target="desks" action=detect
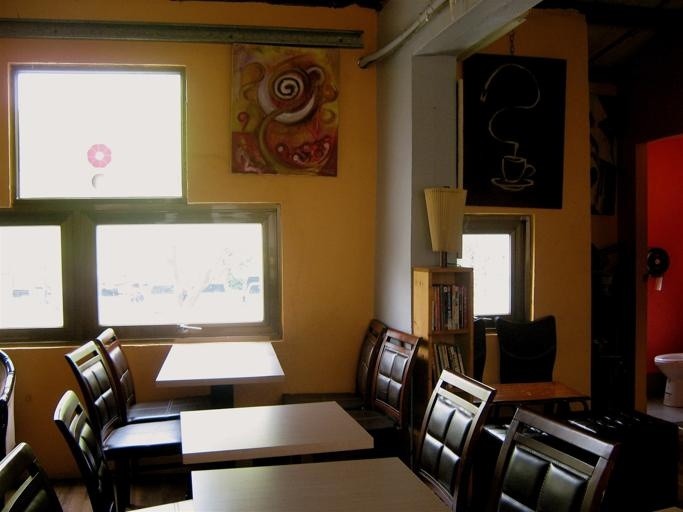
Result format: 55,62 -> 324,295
190,457 -> 450,509
156,339 -> 285,408
180,402 -> 375,500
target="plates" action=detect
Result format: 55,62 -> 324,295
492,176 -> 536,192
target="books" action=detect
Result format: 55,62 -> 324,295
431,282 -> 469,333
431,343 -> 466,390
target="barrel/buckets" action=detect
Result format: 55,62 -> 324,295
654,353 -> 683,408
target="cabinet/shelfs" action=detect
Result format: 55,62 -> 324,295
412,267 -> 476,435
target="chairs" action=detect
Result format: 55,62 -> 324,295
483,408 -> 618,510
0,328 -> 216,512
496,315 -> 592,421
282,322 -> 388,409
411,369 -> 498,510
474,322 -> 487,383
340,328 -> 423,466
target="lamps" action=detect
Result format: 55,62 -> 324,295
424,185 -> 467,269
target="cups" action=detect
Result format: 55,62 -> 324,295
500,154 -> 537,184
